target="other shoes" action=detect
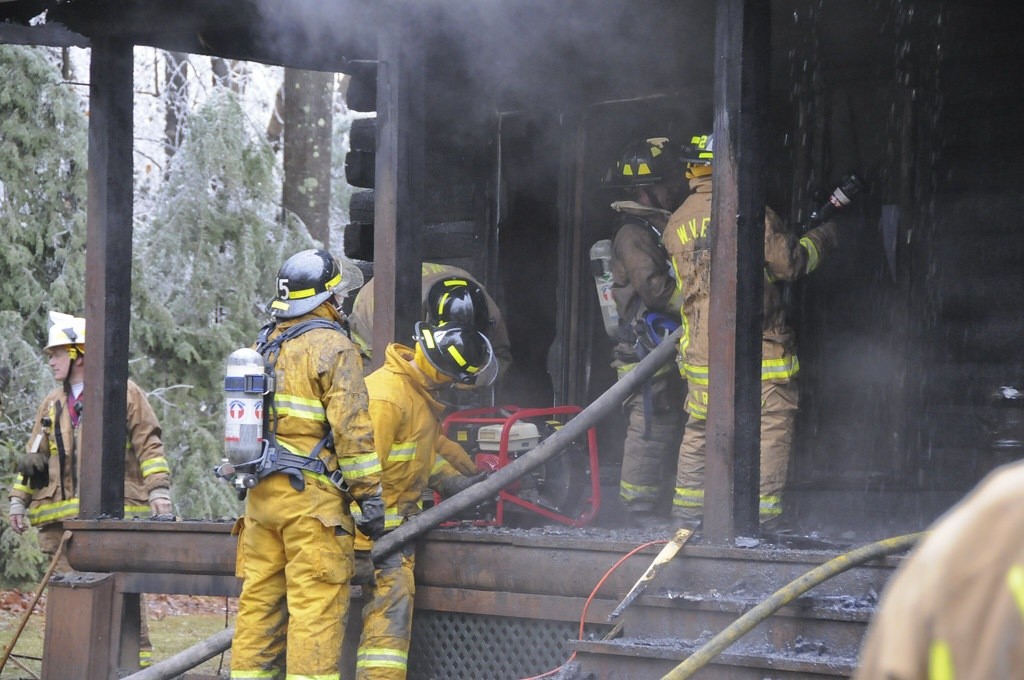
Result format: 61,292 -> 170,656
624,512 -> 676,530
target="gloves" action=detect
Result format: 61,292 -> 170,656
15,454 -> 50,490
436,470 -> 492,515
355,496 -> 385,541
825,207 -> 850,240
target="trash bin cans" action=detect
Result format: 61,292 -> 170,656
984,392 -> 1024,469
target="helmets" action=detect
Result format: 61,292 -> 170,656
413,321 -> 498,388
679,133 -> 714,165
436,276 -> 488,333
596,132 -> 684,189
265,249 -> 364,318
43,317 -> 87,355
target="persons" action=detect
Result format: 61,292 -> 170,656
351,323 -> 498,680
9,310 -> 175,653
214,247 -> 386,680
347,261 -> 512,390
588,143 -> 685,529
660,132 -> 847,534
